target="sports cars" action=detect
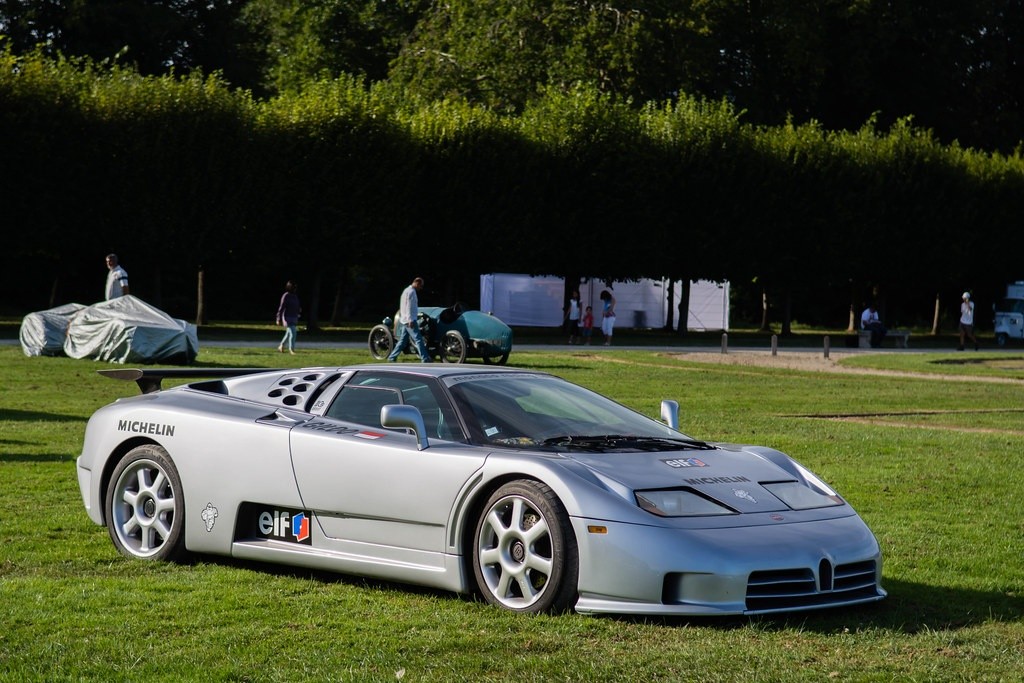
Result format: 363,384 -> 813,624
75,363 -> 889,621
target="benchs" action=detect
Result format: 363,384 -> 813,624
858,330 -> 911,348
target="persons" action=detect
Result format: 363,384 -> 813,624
861,303 -> 887,347
105,254 -> 129,301
565,290 -> 593,345
958,292 -> 981,350
600,290 -> 617,344
386,277 -> 434,362
277,280 -> 302,354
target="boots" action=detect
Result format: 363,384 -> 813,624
575,337 -> 580,344
568,335 -> 573,343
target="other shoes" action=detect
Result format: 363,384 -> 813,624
974,344 -> 978,350
584,342 -> 591,346
278,344 -> 285,353
289,348 -> 296,355
602,342 -> 610,346
958,346 -> 964,350
